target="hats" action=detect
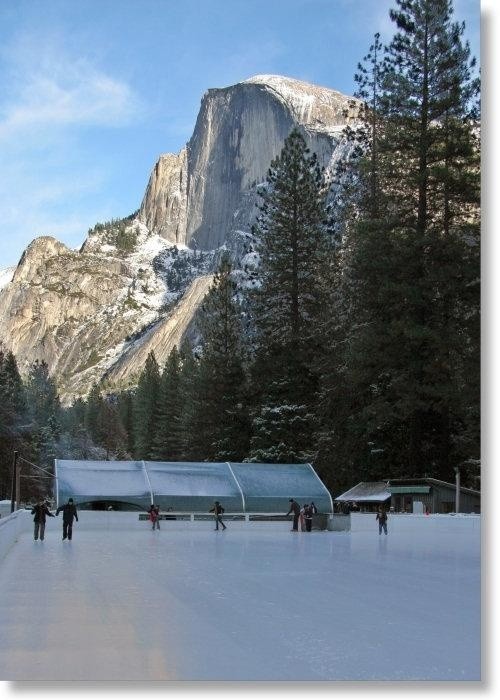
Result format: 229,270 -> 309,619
289,498 -> 293,503
69,498 -> 74,502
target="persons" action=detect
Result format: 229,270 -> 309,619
299,503 -> 308,532
285,499 -> 300,533
309,501 -> 318,514
304,505 -> 313,532
147,503 -> 158,530
30,496 -> 55,542
209,500 -> 227,531
154,503 -> 161,530
55,498 -> 78,541
375,504 -> 388,535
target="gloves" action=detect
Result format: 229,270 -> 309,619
51,513 -> 78,521
